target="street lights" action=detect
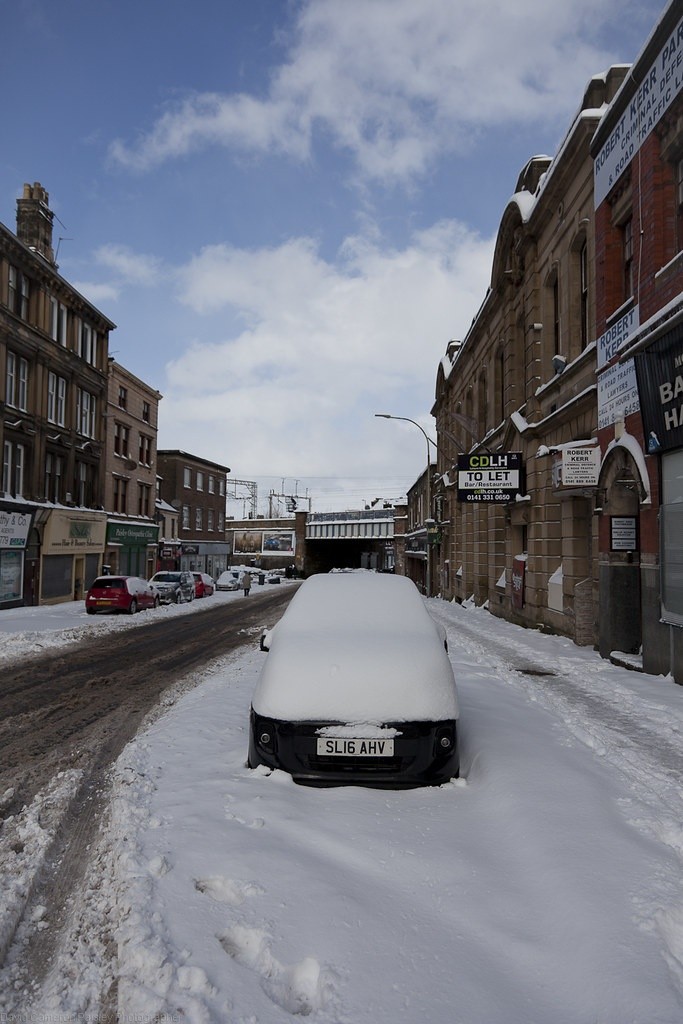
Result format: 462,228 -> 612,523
374,414 -> 431,598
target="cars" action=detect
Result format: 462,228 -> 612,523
149,571 -> 196,604
85,576 -> 161,615
190,572 -> 214,598
247,573 -> 460,791
216,571 -> 245,591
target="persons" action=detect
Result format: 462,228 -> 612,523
242,572 -> 253,596
239,534 -> 255,553
